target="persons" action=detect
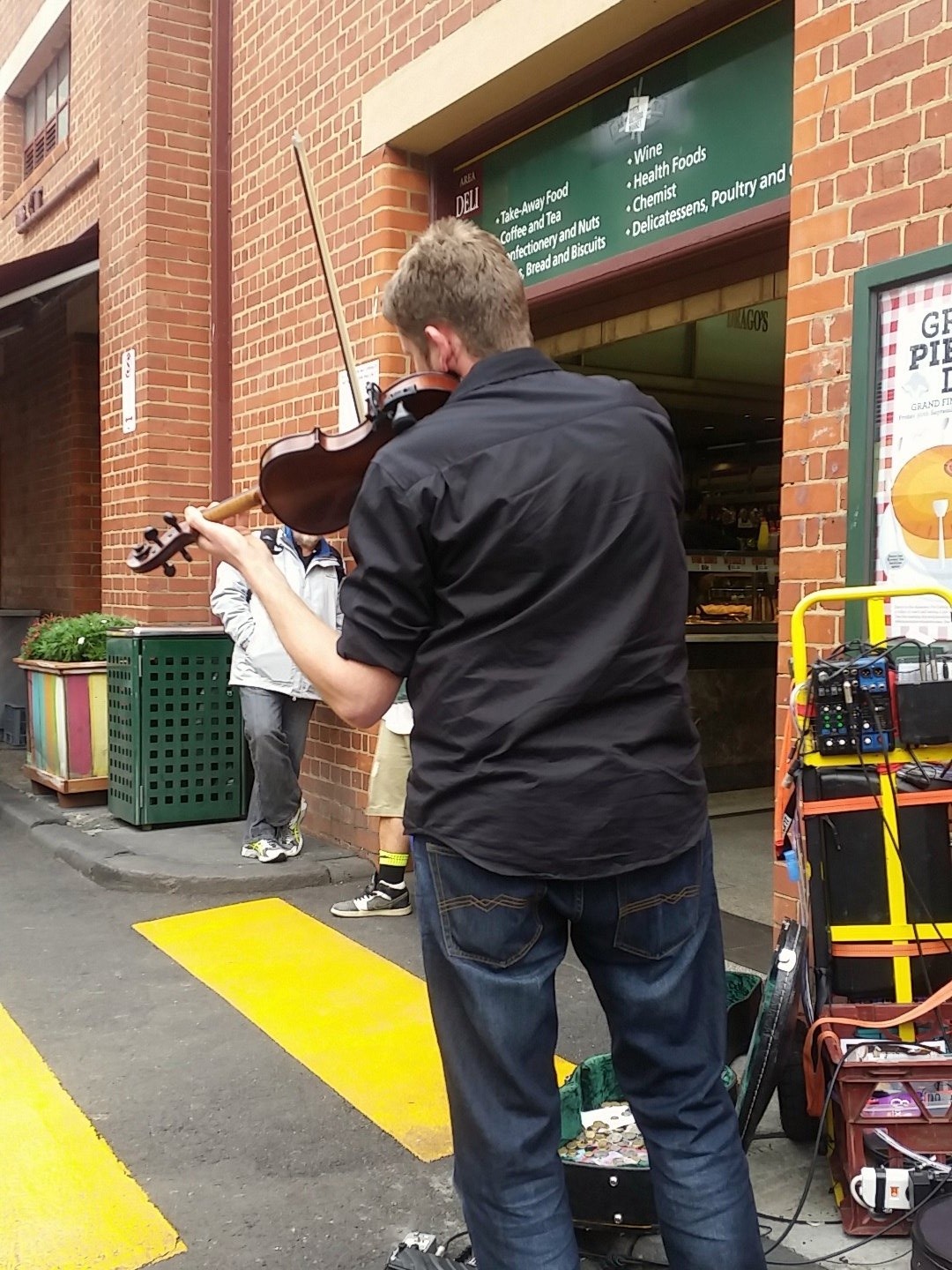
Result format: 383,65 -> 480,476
182,216 -> 768,1270
329,677 -> 416,918
209,522 -> 346,864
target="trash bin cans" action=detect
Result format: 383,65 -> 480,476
106,624 -> 247,830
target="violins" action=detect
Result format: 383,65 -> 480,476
124,371 -> 463,577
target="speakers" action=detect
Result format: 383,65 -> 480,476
800,767 -> 952,1000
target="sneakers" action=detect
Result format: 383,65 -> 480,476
332,874 -> 411,916
278,797 -> 307,856
241,839 -> 287,862
